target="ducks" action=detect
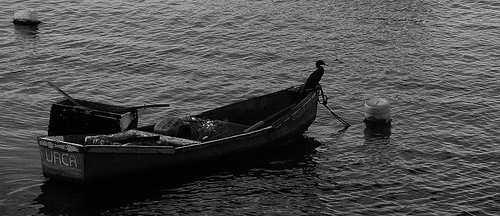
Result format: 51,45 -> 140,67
305,59 -> 329,89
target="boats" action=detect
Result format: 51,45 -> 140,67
37,83 -> 322,185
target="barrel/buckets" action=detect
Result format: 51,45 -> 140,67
365,97 -> 391,129
13,9 -> 37,22
